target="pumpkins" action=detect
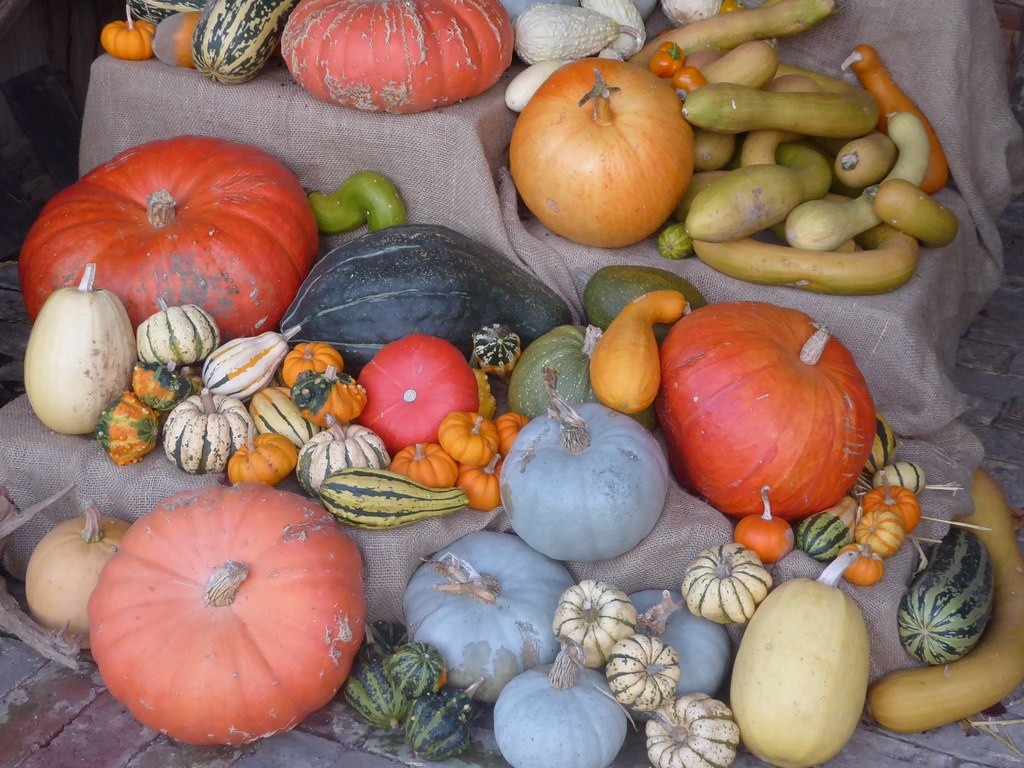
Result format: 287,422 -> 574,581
20,0 -> 1024,768
